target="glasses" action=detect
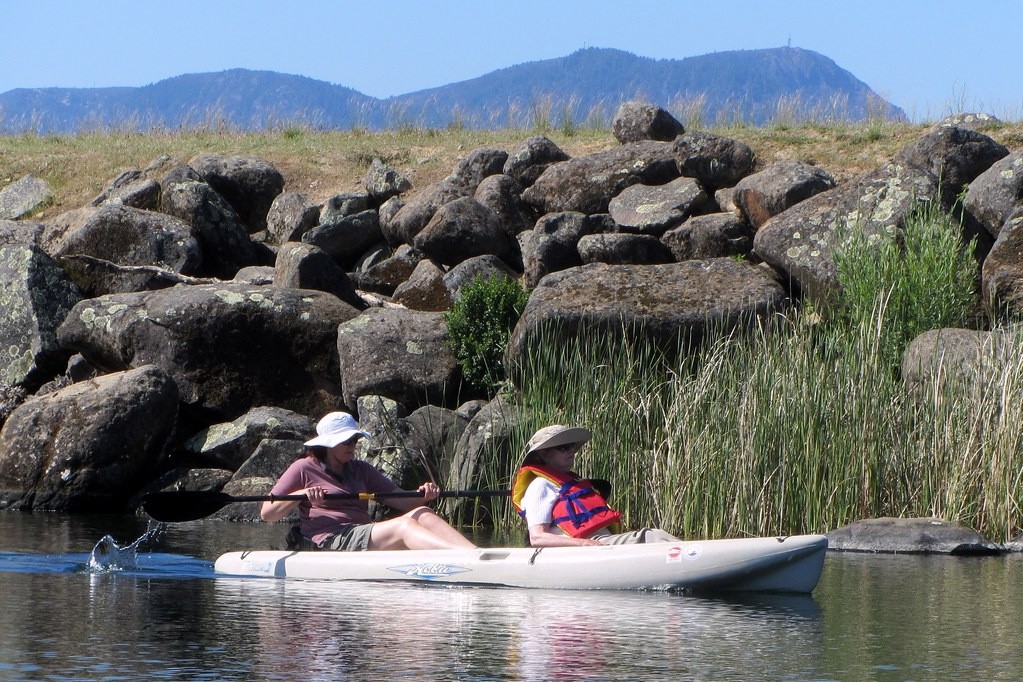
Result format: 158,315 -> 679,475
553,442 -> 577,452
343,435 -> 359,445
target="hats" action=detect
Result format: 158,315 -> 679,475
519,424 -> 593,469
304,410 -> 371,448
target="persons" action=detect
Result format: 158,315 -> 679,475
511,423 -> 683,544
262,412 -> 479,550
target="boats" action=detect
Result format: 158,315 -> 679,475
215,536 -> 828,590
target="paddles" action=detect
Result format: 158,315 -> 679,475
144,476 -> 612,523
